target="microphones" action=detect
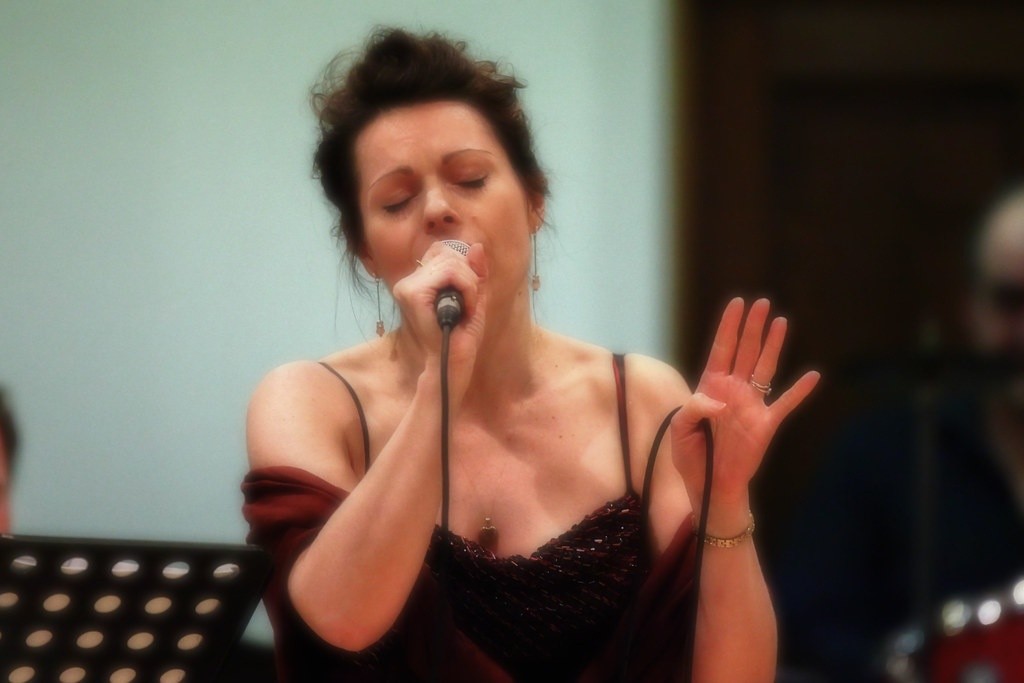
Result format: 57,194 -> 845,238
435,238 -> 470,330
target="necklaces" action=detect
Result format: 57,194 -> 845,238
392,326 -> 543,557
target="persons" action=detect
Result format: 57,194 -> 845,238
886,184 -> 1024,683
0,384 -> 18,536
241,25 -> 821,683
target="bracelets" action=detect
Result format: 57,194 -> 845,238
690,508 -> 755,548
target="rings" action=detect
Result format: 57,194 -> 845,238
751,381 -> 772,396
417,260 -> 425,266
